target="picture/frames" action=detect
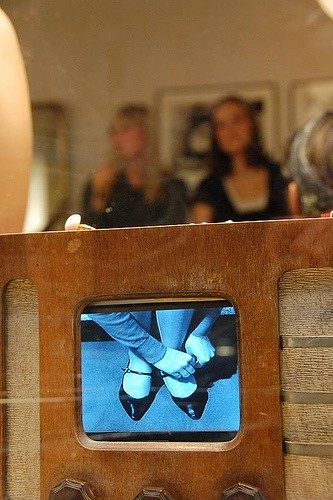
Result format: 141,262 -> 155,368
287,75 -> 333,133
157,81 -> 283,175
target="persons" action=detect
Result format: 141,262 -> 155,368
0,6 -> 33,235
82,103 -> 189,231
192,96 -> 303,222
88,306 -> 222,420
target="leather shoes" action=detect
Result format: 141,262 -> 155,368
159,364 -> 208,421
117,357 -> 152,421
